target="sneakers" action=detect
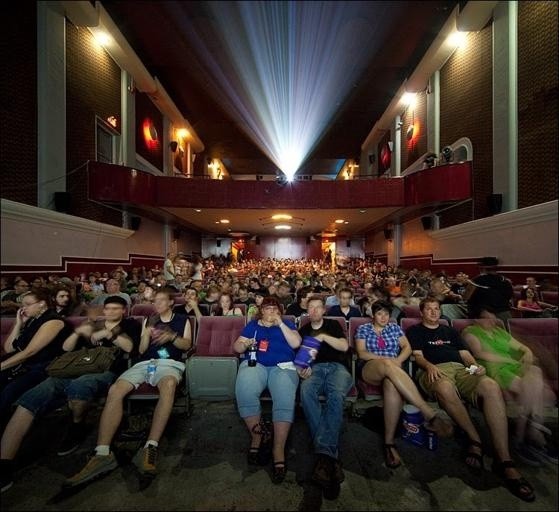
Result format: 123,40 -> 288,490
53,425 -> 85,457
1,472 -> 14,492
272,461 -> 287,485
312,455 -> 334,487
248,447 -> 263,468
332,457 -> 344,485
61,448 -> 119,489
132,444 -> 159,480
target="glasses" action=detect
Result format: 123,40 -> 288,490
20,302 -> 40,310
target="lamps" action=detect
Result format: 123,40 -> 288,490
423,152 -> 437,167
443,145 -> 455,164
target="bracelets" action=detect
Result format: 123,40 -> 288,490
171,334 -> 179,343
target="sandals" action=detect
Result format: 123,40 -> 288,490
464,440 -> 484,473
497,460 -> 534,502
383,443 -> 401,469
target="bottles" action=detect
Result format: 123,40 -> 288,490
150,327 -> 172,360
145,358 -> 157,386
248,343 -> 258,367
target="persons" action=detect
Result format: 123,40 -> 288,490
233,295 -> 352,484
353,300 -> 454,467
1,249 -> 542,332
460,302 -> 558,456
0,288 -> 192,492
406,298 -> 534,496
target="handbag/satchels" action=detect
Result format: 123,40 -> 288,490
251,414 -> 274,465
46,345 -> 121,378
